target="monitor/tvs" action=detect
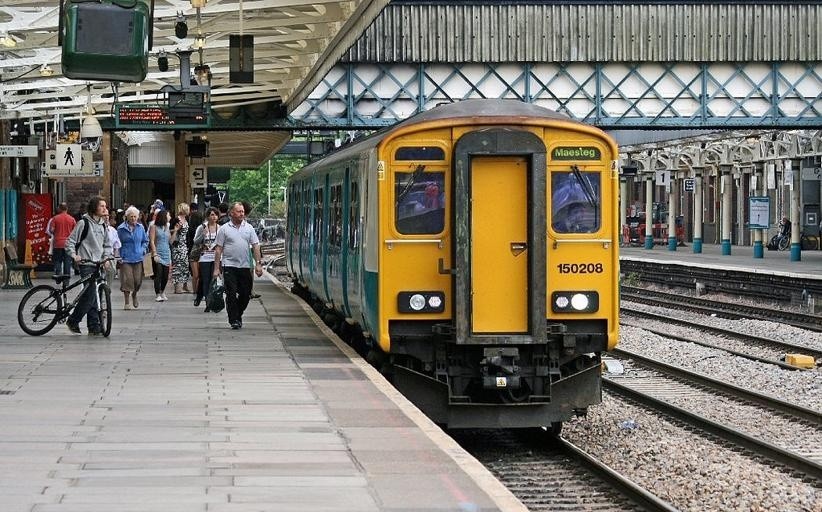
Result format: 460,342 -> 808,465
186,140 -> 209,157
58,0 -> 154,83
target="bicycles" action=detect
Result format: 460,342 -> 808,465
767,223 -> 820,252
16,255 -> 117,336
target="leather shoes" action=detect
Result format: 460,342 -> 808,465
231,319 -> 242,328
193,293 -> 203,306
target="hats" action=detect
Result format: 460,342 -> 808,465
153,201 -> 163,211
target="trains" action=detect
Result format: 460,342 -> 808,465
282,94 -> 622,446
250,217 -> 285,241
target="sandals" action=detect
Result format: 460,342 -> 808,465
249,293 -> 261,299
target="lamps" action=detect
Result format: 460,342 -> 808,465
80,81 -> 103,140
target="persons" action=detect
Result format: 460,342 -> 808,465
782,216 -> 791,247
45,196 -> 264,329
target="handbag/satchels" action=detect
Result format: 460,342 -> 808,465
205,273 -> 225,314
141,247 -> 154,278
172,223 -> 183,248
190,223 -> 207,262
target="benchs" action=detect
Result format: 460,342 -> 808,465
3,242 -> 35,292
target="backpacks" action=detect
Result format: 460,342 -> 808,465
72,217 -> 107,271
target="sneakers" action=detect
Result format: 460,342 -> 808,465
123,304 -> 132,310
132,293 -> 139,308
88,325 -> 105,338
66,316 -> 82,335
155,293 -> 169,302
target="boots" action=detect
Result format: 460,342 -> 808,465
174,282 -> 194,294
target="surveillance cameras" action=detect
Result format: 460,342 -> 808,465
82,117 -> 103,141
